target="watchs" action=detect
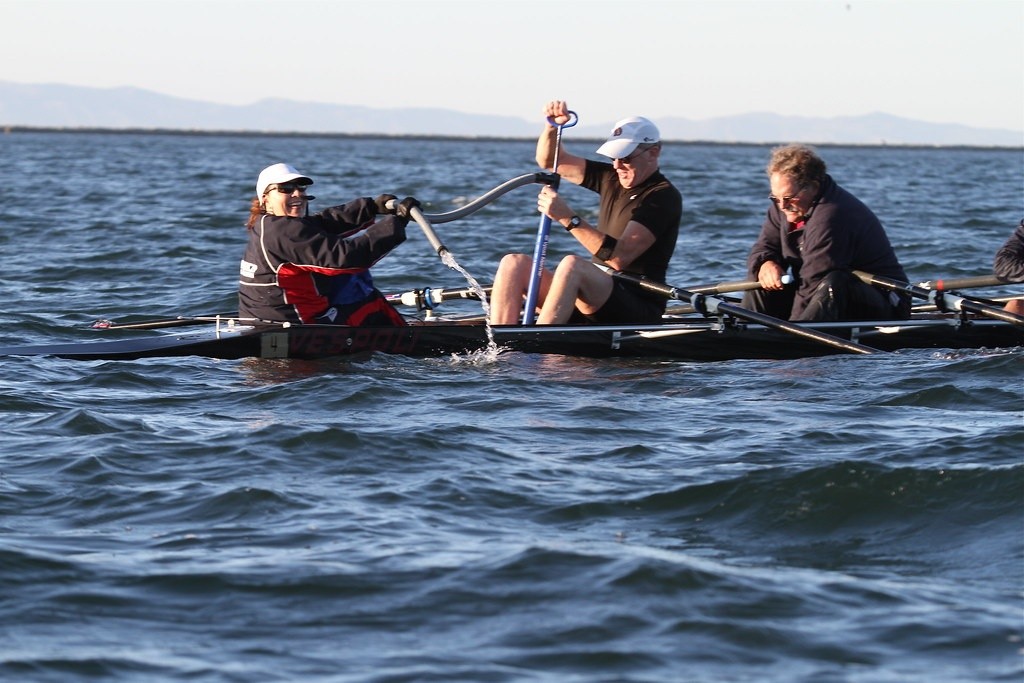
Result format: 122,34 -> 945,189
563,216 -> 581,231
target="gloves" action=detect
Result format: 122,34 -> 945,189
395,196 -> 424,228
374,193 -> 398,214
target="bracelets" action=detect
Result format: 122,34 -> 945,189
593,234 -> 618,261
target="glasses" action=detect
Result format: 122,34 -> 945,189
610,145 -> 658,163
264,181 -> 308,196
768,181 -> 810,204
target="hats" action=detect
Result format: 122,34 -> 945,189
596,116 -> 660,159
257,163 -> 314,202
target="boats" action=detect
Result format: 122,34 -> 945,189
0,286 -> 1024,362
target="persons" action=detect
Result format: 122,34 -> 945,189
489,100 -> 683,327
739,145 -> 912,323
993,218 -> 1024,316
238,162 -> 424,328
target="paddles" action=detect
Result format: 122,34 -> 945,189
905,276 -> 1024,291
671,271 -> 796,294
592,262 -> 881,354
90,285 -> 493,331
851,270 -> 1024,326
